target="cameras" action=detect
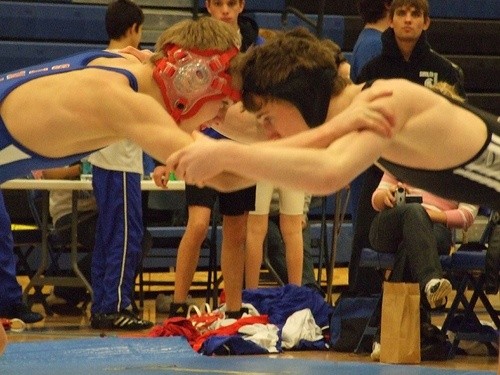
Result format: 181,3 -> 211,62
393,186 -> 423,205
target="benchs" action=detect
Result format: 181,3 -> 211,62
0,1 -> 500,283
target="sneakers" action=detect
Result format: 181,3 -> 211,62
371,343 -> 381,360
424,278 -> 452,308
16,313 -> 42,323
90,312 -> 153,330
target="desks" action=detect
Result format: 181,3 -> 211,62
0,178 -> 221,314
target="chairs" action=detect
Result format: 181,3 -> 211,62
437,208 -> 500,361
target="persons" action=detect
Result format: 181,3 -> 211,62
42,160 -> 153,316
0,17 -> 397,353
153,0 -> 267,321
120,37 -> 500,213
88,0 -> 154,329
238,16 -> 351,300
323,0 -> 465,308
370,82 -> 479,360
